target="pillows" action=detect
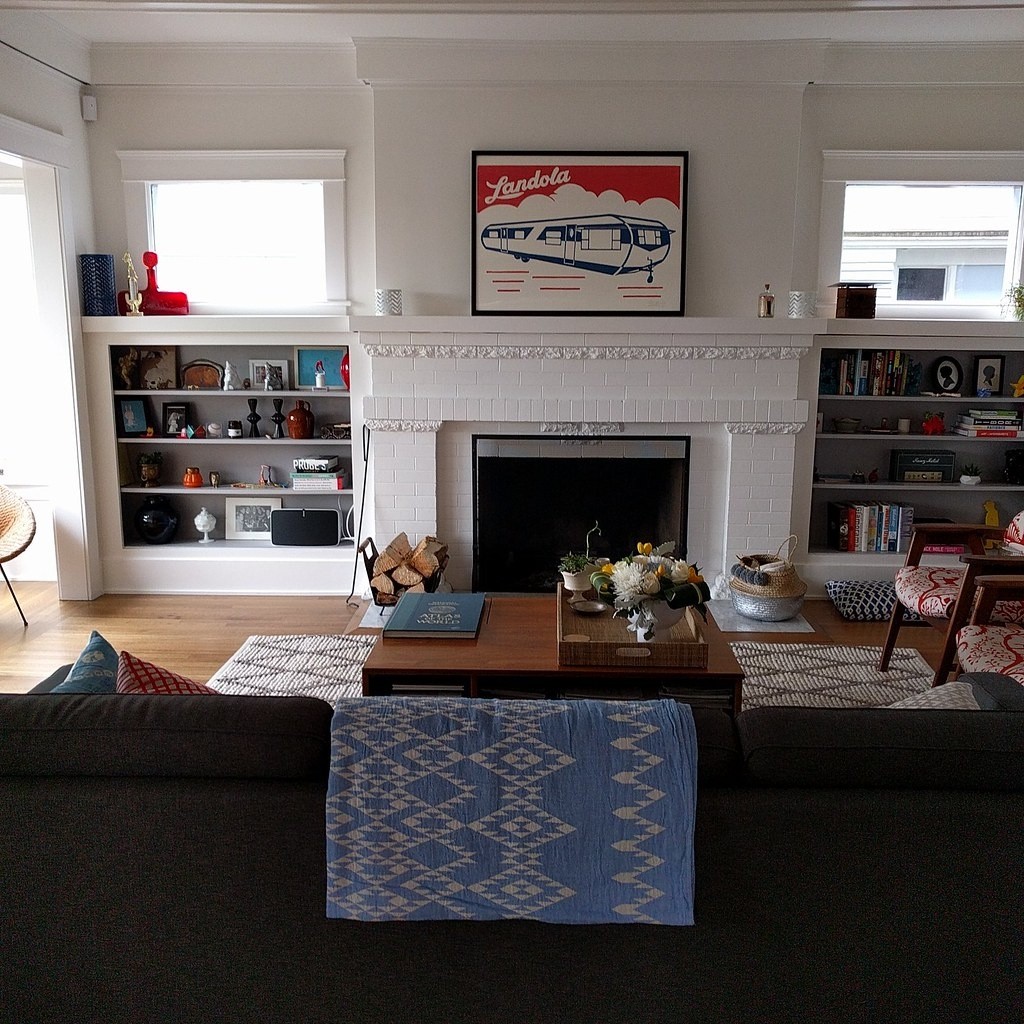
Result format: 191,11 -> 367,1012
117,651 -> 219,694
825,579 -> 924,621
49,630 -> 118,693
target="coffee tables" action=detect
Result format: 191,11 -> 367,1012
363,597 -> 745,710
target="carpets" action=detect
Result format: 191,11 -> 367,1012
207,635 -> 933,709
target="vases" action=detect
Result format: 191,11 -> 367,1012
627,601 -> 685,642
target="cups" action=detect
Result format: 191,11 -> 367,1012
898,419 -> 910,432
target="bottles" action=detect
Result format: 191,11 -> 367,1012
758,283 -> 775,318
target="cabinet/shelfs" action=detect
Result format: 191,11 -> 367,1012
115,389 -> 353,494
812,395 -> 1024,492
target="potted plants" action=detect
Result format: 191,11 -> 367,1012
557,551 -> 596,591
960,463 -> 985,486
923,409 -> 947,435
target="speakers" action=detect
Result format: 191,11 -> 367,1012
271,510 -> 343,547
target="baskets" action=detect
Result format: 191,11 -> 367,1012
729,535 -> 808,623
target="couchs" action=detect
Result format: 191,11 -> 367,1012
0,663 -> 1024,1024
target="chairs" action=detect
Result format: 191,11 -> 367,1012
878,511 -> 1024,687
0,483 -> 37,626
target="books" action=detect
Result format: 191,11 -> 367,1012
826,500 -> 914,553
390,682 -> 734,711
839,349 -> 922,397
382,592 -> 486,640
949,409 -> 1024,438
922,543 -> 965,554
289,454 -> 350,491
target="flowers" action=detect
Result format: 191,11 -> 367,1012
592,542 -> 711,640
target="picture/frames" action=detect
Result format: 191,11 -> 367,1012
471,151 -> 689,317
293,346 -> 348,390
972,354 -> 1006,396
930,356 -> 964,394
161,401 -> 190,438
248,359 -> 289,388
225,497 -> 281,541
114,395 -> 157,438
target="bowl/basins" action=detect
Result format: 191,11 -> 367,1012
569,601 -> 608,617
